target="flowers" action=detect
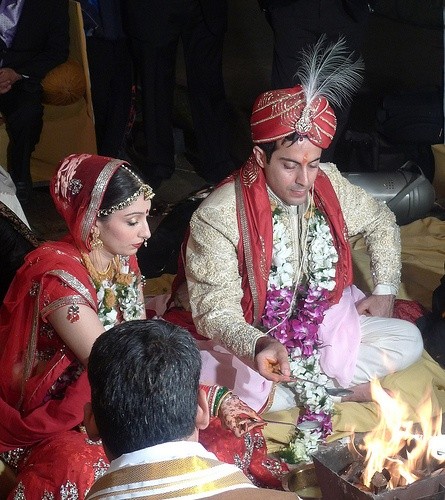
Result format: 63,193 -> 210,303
267,200 -> 339,470
98,266 -> 146,325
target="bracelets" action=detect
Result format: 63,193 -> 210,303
208,384 -> 234,418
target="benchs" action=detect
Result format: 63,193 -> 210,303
0,0 -> 96,193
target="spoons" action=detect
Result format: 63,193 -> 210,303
291,376 -> 355,396
254,419 -> 321,430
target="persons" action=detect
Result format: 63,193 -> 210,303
162,86 -> 423,416
257,0 -> 377,173
0,0 -> 70,206
84,319 -> 304,500
73,0 -> 243,193
0,154 -> 269,500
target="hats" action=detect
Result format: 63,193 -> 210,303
250,31 -> 367,149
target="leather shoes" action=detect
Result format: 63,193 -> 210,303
6,165 -> 31,190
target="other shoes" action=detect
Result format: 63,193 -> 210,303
140,164 -> 173,191
199,162 -> 240,183
